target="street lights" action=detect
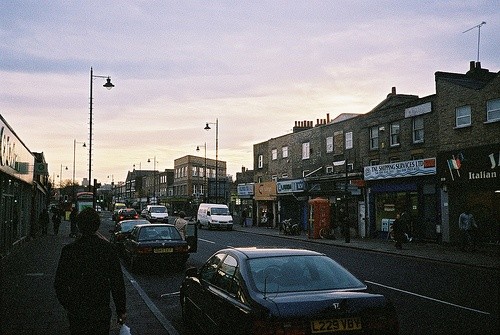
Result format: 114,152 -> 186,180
147,155 -> 156,206
86,66 -> 115,191
107,174 -> 114,199
73,139 -> 87,186
204,118 -> 219,203
133,161 -> 143,190
195,142 -> 207,203
53,172 -> 60,189
60,164 -> 69,189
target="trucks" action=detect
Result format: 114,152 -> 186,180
146,205 -> 169,223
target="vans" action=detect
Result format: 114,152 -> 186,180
96,206 -> 101,213
197,203 -> 234,231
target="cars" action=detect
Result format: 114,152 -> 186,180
115,208 -> 139,223
114,203 -> 128,213
179,245 -> 399,335
123,223 -> 199,275
109,219 -> 151,250
141,208 -> 147,217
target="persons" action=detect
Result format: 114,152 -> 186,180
339,207 -> 346,240
174,212 -> 188,241
391,215 -> 405,251
55,207 -> 126,335
459,208 -> 477,254
40,209 -> 49,235
241,208 -> 247,228
52,211 -> 61,236
266,210 -> 273,229
69,208 -> 77,237
